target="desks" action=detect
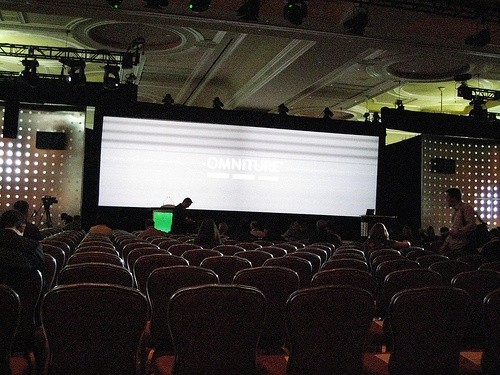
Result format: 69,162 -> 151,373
361,215 -> 397,237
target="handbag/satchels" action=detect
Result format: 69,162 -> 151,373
463,221 -> 490,250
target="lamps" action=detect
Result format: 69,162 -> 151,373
278,103 -> 289,115
212,97 -> 224,108
363,112 -> 371,122
103,64 -> 120,90
20,56 -> 39,82
161,93 -> 174,104
106,0 -> 369,36
322,107 -> 334,119
395,100 -> 404,110
68,60 -> 86,86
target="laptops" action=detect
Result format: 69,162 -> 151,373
366,209 -> 374,215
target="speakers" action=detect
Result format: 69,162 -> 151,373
36,131 -> 66,150
432,159 -> 455,174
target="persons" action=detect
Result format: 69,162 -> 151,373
441,188 -> 476,256
369,222 -> 411,249
219,222 -> 228,235
194,219 -> 221,244
0,209 -> 45,326
402,225 -> 500,240
284,219 -> 341,244
14,201 -> 44,240
171,198 -> 196,234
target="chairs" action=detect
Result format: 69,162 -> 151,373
0,226 -> 500,375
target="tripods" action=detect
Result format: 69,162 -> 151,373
39,206 -> 52,228
